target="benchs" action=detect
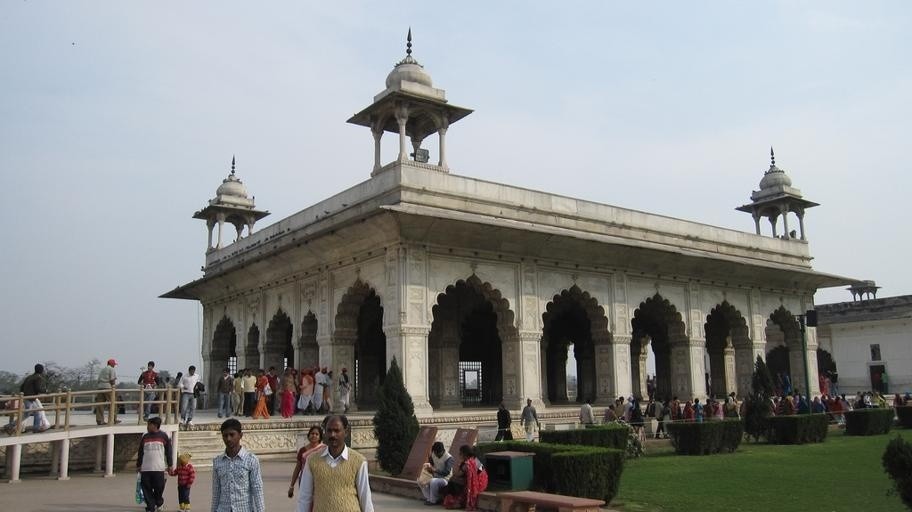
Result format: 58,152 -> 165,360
494,491 -> 607,511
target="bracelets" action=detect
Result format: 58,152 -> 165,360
289,485 -> 294,489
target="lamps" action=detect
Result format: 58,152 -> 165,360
789,230 -> 796,240
409,148 -> 431,163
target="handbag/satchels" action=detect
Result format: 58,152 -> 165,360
134,470 -> 144,504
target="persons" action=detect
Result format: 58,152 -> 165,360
211,418 -> 264,509
288,425 -> 322,498
493,401 -> 513,441
296,415 -> 374,509
336,368 -> 352,414
176,372 -> 182,385
443,444 -> 489,511
216,368 -> 234,417
138,362 -> 160,421
580,369 -> 912,445
280,367 -> 334,419
417,441 -> 454,506
520,397 -> 539,443
3,364 -> 47,436
230,366 -> 278,418
169,453 -> 196,508
137,417 -> 173,509
177,366 -> 202,425
95,361 -> 121,425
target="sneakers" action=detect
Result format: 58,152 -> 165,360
96,420 -> 122,425
156,503 -> 165,512
179,503 -> 191,512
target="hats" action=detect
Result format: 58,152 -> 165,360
148,417 -> 161,425
179,453 -> 192,465
107,358 -> 117,367
